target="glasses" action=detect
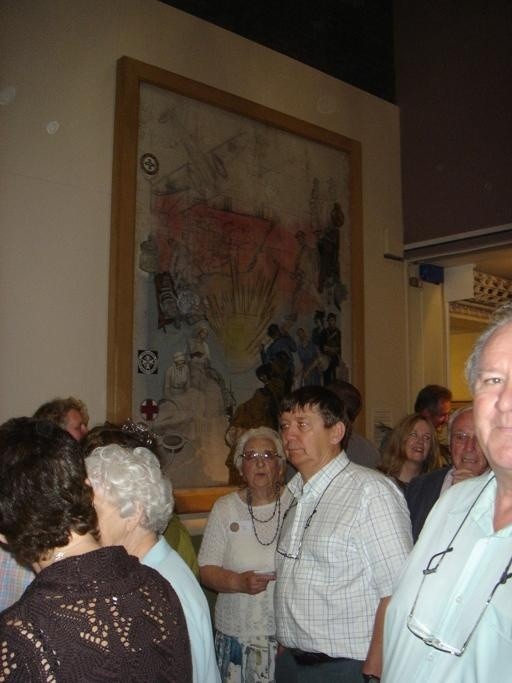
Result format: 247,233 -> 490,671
405,547 -> 512,657
276,503 -> 317,560
241,450 -> 278,464
449,430 -> 478,446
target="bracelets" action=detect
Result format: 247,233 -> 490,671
362,673 -> 380,683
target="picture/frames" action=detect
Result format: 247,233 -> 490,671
103,56 -> 368,517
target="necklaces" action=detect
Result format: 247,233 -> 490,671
248,486 -> 280,545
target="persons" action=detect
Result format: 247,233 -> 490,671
381,316 -> 512,683
0,396 -> 221,683
195,425 -> 292,683
270,385 -> 414,683
340,382 -> 487,544
